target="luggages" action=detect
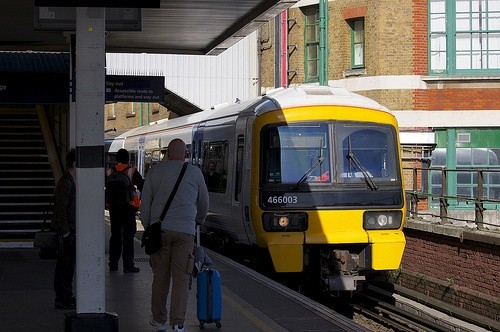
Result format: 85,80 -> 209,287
195,221 -> 222,329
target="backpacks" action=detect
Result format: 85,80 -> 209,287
105,165 -> 134,206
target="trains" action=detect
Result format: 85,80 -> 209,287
109,81 -> 408,308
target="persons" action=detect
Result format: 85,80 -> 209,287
106,149 -> 145,274
203,162 -> 220,193
140,139 -> 209,332
50,149 -> 77,310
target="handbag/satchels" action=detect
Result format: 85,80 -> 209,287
141,222 -> 162,255
35,229 -> 64,260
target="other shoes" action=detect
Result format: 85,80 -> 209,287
171,324 -> 185,332
55,299 -> 75,310
151,318 -> 168,331
110,266 -> 118,271
123,267 -> 140,273
